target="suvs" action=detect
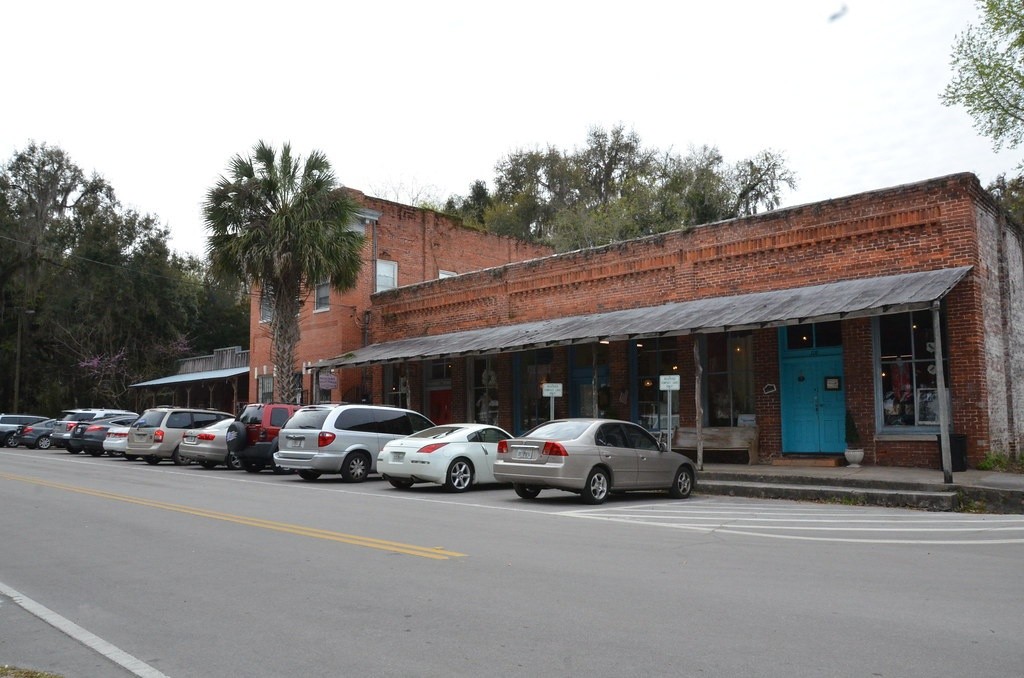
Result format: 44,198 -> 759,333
125,405 -> 236,465
226,401 -> 307,475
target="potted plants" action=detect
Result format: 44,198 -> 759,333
844,408 -> 865,468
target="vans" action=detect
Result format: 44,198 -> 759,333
0,414 -> 50,448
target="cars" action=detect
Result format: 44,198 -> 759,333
103,416 -> 161,463
13,418 -> 58,449
493,418 -> 699,505
68,416 -> 124,457
179,417 -> 242,471
53,407 -> 140,454
377,423 -> 514,493
273,401 -> 436,483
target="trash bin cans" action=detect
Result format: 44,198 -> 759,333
936,432 -> 968,472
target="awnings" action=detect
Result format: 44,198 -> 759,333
304,266 -> 971,371
129,366 -> 249,387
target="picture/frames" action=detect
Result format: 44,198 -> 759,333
916,387 -> 950,425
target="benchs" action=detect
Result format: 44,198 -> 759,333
671,425 -> 760,467
598,433 -> 617,446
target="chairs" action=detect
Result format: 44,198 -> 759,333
630,435 -> 641,448
